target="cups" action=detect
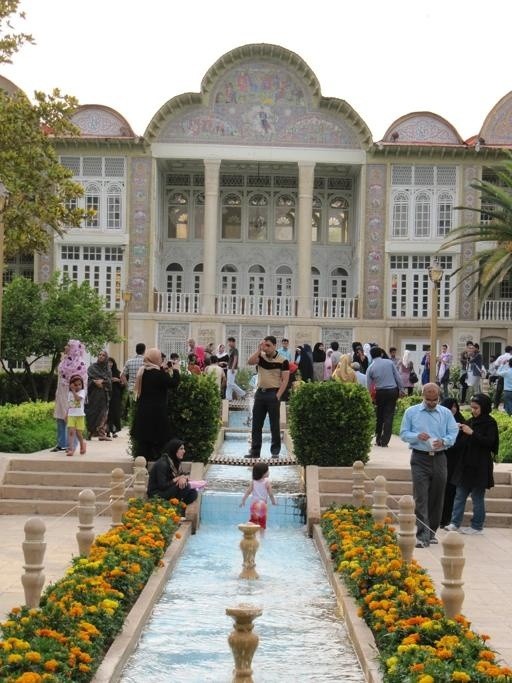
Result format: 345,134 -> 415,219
428,437 -> 438,448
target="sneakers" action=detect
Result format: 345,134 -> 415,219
416,523 -> 484,547
50,434 -> 118,455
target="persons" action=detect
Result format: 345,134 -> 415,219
147,438 -> 198,516
441,400 -> 467,531
399,382 -> 459,550
445,394 -> 499,535
52,336 -> 247,458
186,67 -> 304,136
237,463 -> 277,538
243,336 -> 512,459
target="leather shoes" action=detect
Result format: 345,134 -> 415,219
244,453 -> 260,457
272,453 -> 279,457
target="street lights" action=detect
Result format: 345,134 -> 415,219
427,254 -> 444,383
122,283 -> 134,367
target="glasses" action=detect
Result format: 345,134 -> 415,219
425,397 -> 439,403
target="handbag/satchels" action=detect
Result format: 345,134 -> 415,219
481,365 -> 487,378
410,372 -> 418,382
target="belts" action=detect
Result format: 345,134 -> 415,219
258,387 -> 278,392
413,448 -> 445,455
